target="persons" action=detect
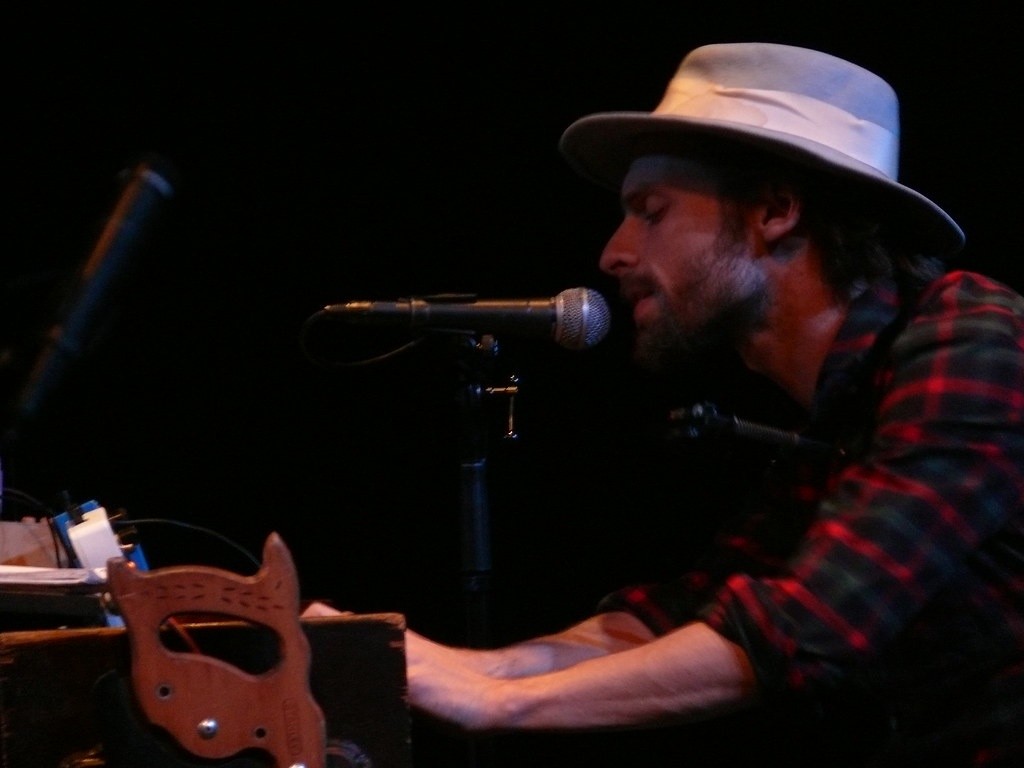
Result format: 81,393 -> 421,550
297,38 -> 1023,768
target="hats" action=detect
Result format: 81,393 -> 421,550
555,41 -> 966,255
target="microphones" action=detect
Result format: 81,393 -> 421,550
322,285 -> 610,352
